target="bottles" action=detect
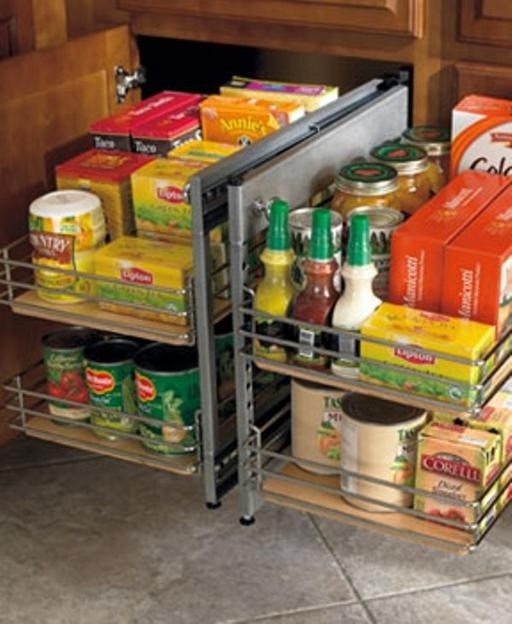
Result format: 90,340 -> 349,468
293,209 -> 340,369
328,213 -> 383,376
253,200 -> 299,364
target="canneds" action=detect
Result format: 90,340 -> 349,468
286,207 -> 344,312
292,377 -> 351,475
331,162 -> 402,260
133,343 -> 200,455
338,393 -> 428,514
401,127 -> 452,196
367,143 -> 430,221
81,338 -> 140,441
346,206 -> 405,302
41,327 -> 100,424
